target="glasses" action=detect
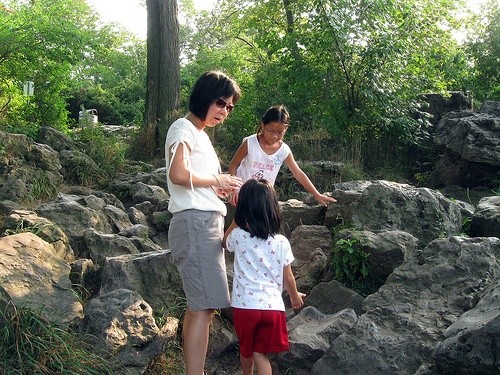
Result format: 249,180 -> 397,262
265,125 -> 286,136
215,98 -> 234,114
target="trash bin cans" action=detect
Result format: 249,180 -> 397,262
79,109 -> 97,127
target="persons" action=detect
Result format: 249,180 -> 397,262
165,71 -> 242,375
223,179 -> 306,375
227,106 -> 337,207
